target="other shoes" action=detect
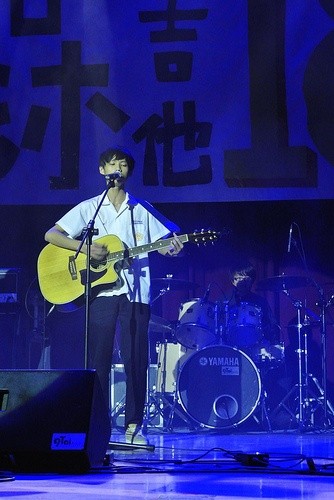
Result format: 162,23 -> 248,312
125,424 -> 149,445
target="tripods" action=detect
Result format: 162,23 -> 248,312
259,288 -> 334,433
110,329 -> 195,434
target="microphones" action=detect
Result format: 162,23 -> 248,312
105,171 -> 121,180
221,398 -> 230,411
287,224 -> 293,252
200,282 -> 212,304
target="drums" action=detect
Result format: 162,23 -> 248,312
257,335 -> 294,371
153,341 -> 193,396
174,297 -> 218,347
174,345 -> 262,431
223,303 -> 268,346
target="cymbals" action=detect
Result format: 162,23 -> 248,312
148,314 -> 172,333
149,276 -> 199,293
259,273 -> 307,291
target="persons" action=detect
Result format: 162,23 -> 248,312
41,149 -> 184,445
228,258 -> 281,344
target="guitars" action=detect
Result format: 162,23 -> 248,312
36,228 -> 221,313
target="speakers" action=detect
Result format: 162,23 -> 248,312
0,369 -> 113,473
